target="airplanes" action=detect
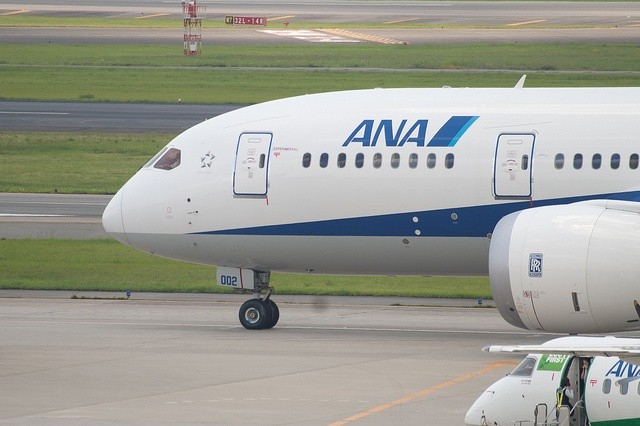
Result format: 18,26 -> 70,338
463,337 -> 640,426
100,73 -> 640,335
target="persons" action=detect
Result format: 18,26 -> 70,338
580,358 -> 590,383
561,377 -> 574,417
